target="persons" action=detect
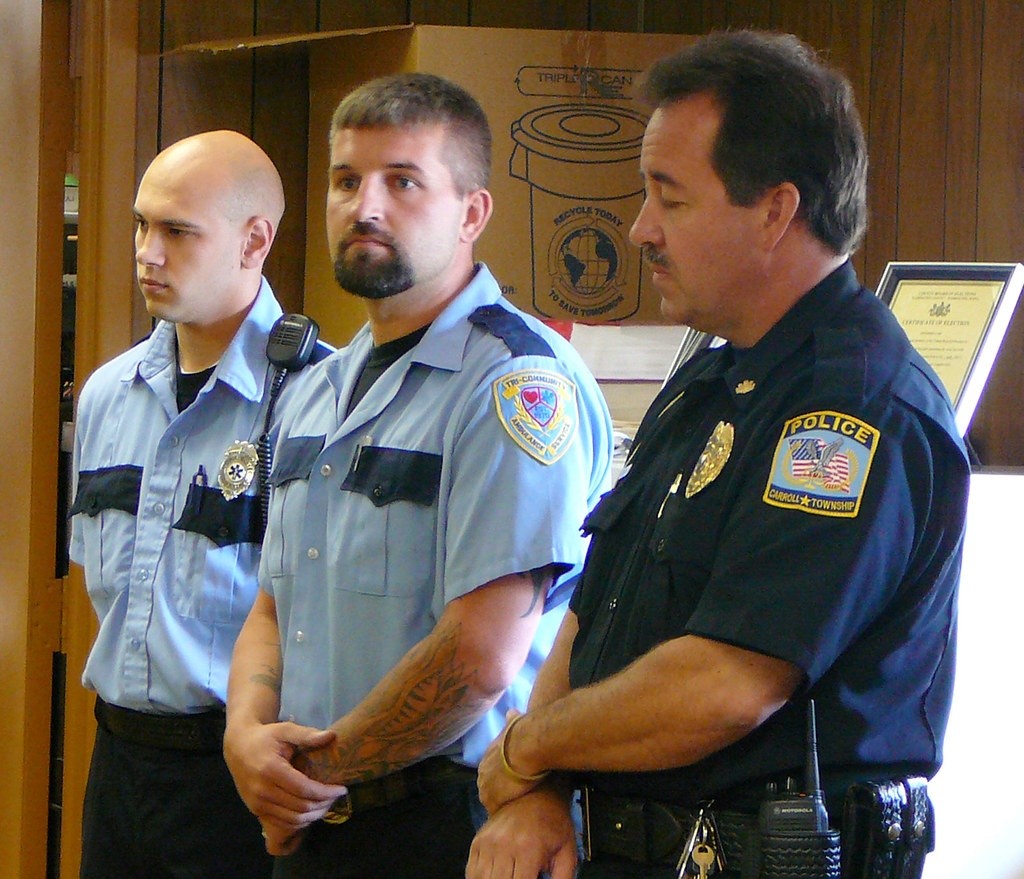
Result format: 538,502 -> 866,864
464,28 -> 970,879
222,71 -> 614,879
67,131 -> 341,879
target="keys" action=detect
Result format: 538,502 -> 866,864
676,800 -> 726,879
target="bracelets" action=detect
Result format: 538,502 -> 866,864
501,715 -> 550,780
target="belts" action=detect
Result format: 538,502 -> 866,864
570,783 -> 763,877
319,773 -> 479,820
94,694 -> 226,754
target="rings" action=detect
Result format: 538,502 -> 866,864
261,829 -> 269,839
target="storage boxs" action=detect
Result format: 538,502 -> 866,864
154,20 -> 788,348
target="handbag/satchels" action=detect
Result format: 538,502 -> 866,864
759,828 -> 841,879
